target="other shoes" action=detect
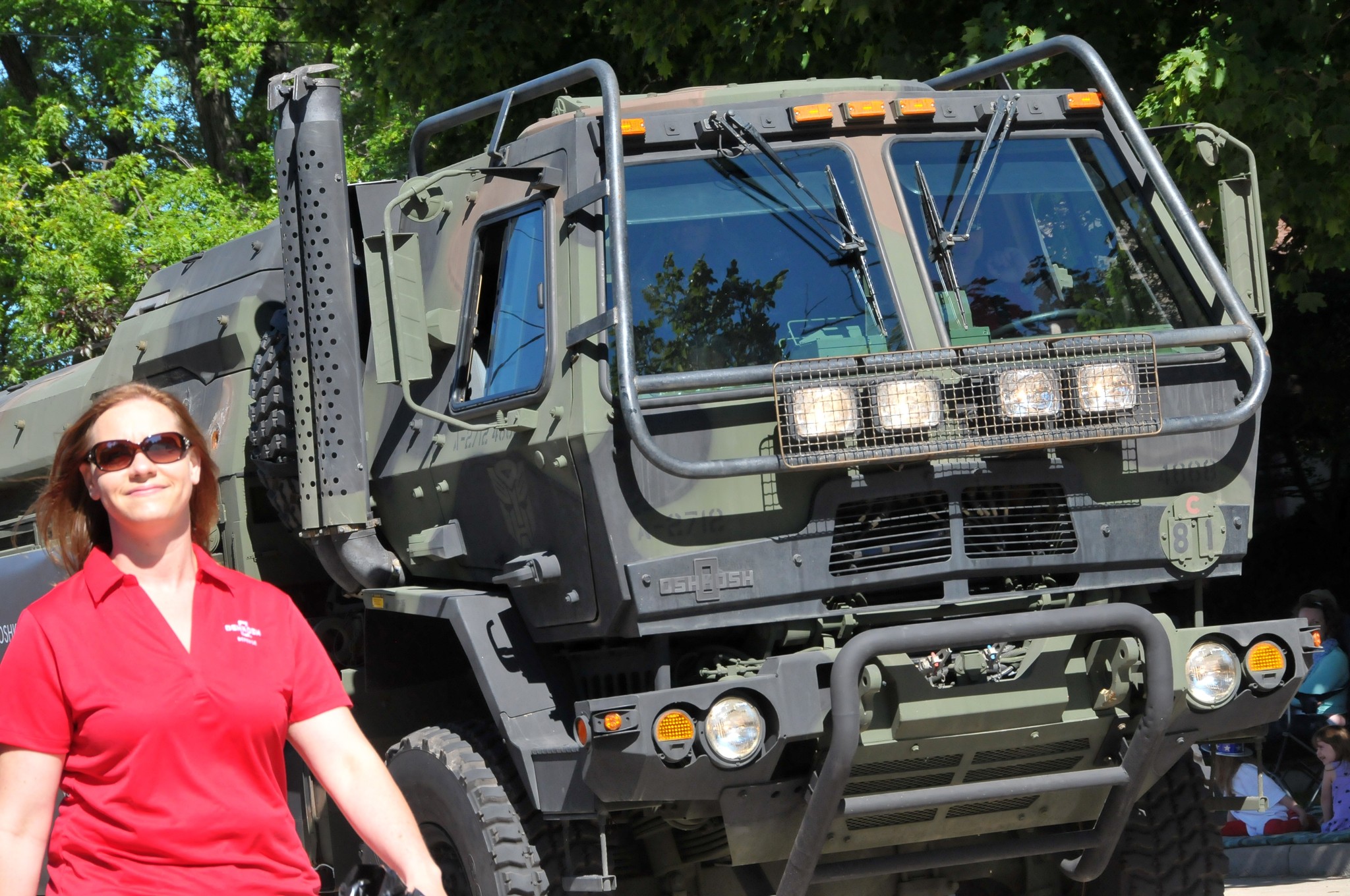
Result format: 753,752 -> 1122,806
1307,815 -> 1321,833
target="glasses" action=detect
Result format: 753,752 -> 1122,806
82,432 -> 190,472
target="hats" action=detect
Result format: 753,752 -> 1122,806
1200,742 -> 1253,757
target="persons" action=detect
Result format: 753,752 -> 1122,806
1199,741 -> 1319,840
1312,725 -> 1350,833
1277,588 -> 1350,817
0,385 -> 450,896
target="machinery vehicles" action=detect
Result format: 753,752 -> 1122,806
0,29 -> 1327,896
